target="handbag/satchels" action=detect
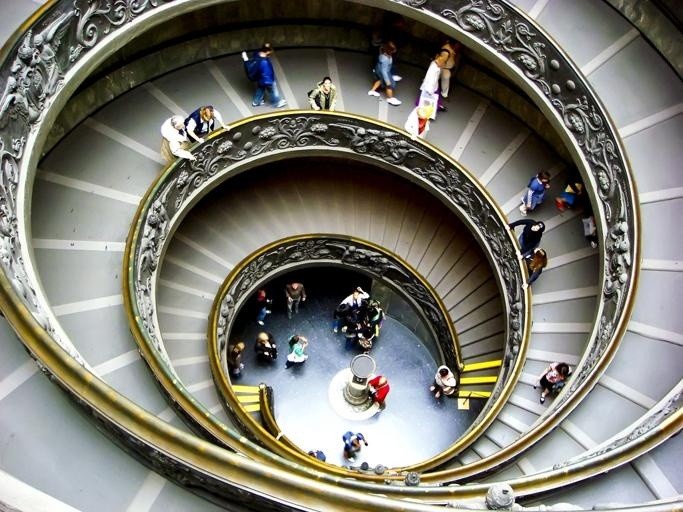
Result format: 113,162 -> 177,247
286,353 -> 304,363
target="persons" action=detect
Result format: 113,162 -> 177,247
343,431 -> 367,461
30,34 -> 54,74
228,280 -> 385,379
365,29 -> 460,139
364,375 -> 390,412
160,115 -> 195,161
310,450 -> 326,461
252,42 -> 286,107
184,104 -> 231,144
308,77 -> 337,111
429,364 -> 457,400
508,166 -> 599,290
534,362 -> 572,404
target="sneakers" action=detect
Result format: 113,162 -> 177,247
368,90 -> 380,96
386,96 -> 402,106
266,310 -> 271,314
252,100 -> 265,106
258,321 -> 264,325
334,327 -> 338,333
276,100 -> 287,108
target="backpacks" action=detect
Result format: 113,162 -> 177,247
245,55 -> 260,83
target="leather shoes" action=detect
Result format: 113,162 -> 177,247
540,392 -> 545,404
